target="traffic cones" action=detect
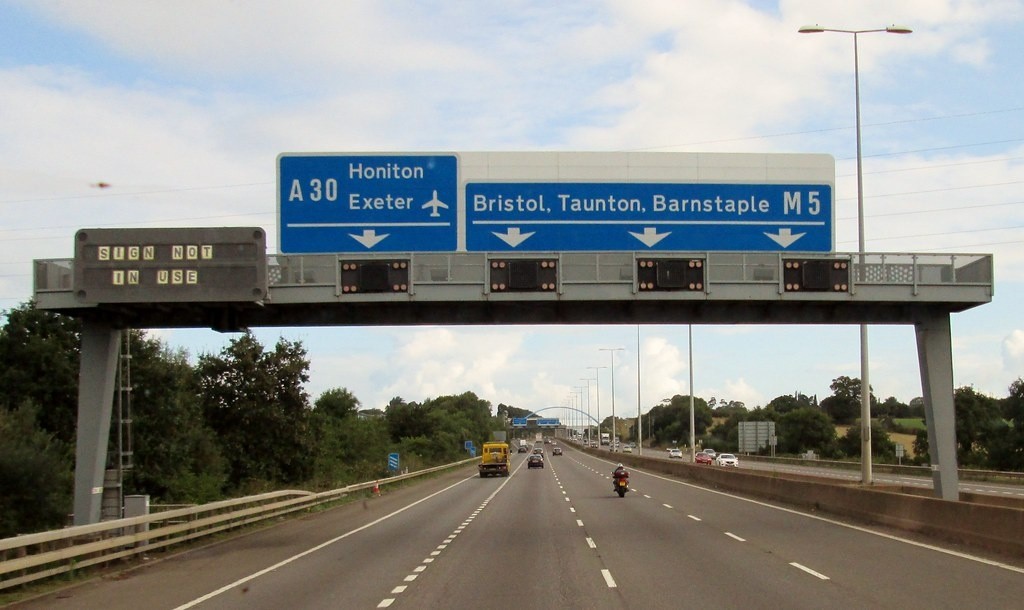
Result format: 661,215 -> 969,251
372,480 -> 380,493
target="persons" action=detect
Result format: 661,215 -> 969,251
613,463 -> 629,492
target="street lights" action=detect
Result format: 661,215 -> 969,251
571,391 -> 582,446
580,378 -> 596,446
799,23 -> 914,483
600,348 -> 625,452
574,386 -> 586,445
586,366 -> 608,449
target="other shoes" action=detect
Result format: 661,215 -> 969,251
614,489 -> 617,491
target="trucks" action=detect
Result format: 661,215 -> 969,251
600,432 -> 620,452
478,440 -> 512,478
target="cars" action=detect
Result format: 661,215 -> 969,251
623,445 -> 632,453
668,449 -> 683,458
694,452 -> 713,466
570,433 -> 598,448
527,454 -> 544,469
702,449 -> 717,460
629,442 -> 636,448
517,432 -> 558,454
533,448 -> 544,458
715,453 -> 739,468
553,448 -> 562,456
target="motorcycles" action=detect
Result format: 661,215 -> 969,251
610,468 -> 628,497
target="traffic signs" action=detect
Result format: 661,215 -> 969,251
459,175 -> 834,253
275,152 -> 459,255
